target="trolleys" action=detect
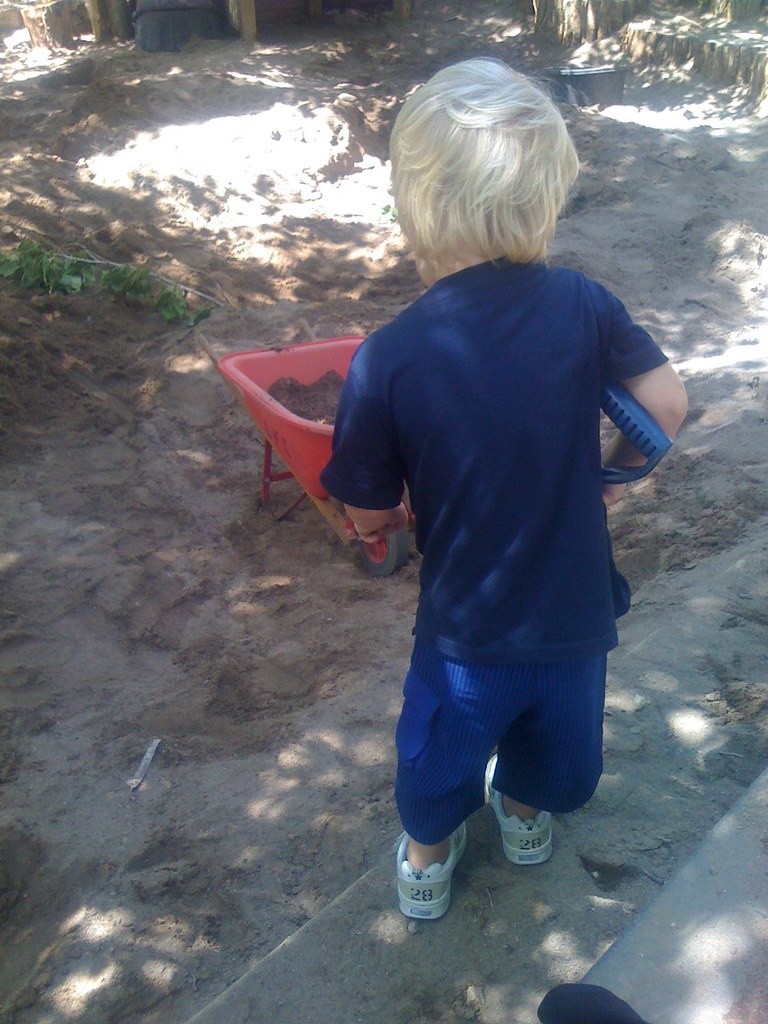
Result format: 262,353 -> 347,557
189,313 -> 429,580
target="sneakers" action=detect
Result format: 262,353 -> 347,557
392,820 -> 467,919
483,753 -> 552,865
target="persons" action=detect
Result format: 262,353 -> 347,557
320,58 -> 687,919
